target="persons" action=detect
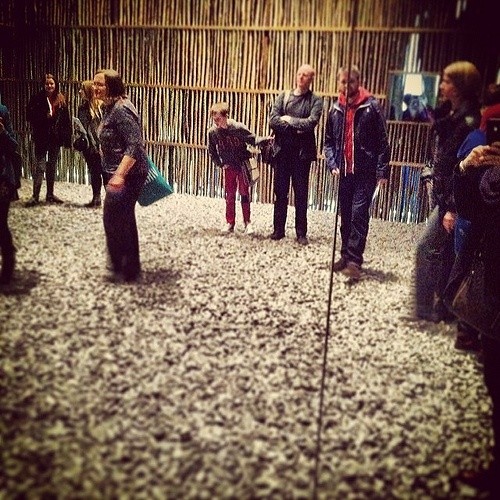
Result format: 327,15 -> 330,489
0,103 -> 40,295
26,74 -> 73,207
267,64 -> 323,246
207,103 -> 274,235
74,80 -> 112,208
324,65 -> 391,281
406,62 -> 500,500
92,69 -> 149,283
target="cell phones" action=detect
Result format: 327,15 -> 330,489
485,117 -> 500,148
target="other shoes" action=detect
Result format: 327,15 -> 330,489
84,199 -> 101,208
475,348 -> 486,367
271,230 -> 286,240
332,258 -> 348,272
398,309 -> 434,321
106,263 -> 114,271
453,337 -> 480,354
26,196 -> 40,207
104,271 -> 134,282
46,194 -> 63,204
345,266 -> 359,284
297,235 -> 308,245
228,224 -> 234,231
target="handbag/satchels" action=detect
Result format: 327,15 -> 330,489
241,158 -> 260,187
73,132 -> 87,151
261,134 -> 282,163
138,156 -> 173,207
448,258 -> 500,342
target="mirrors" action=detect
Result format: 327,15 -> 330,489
384,70 -> 442,126
